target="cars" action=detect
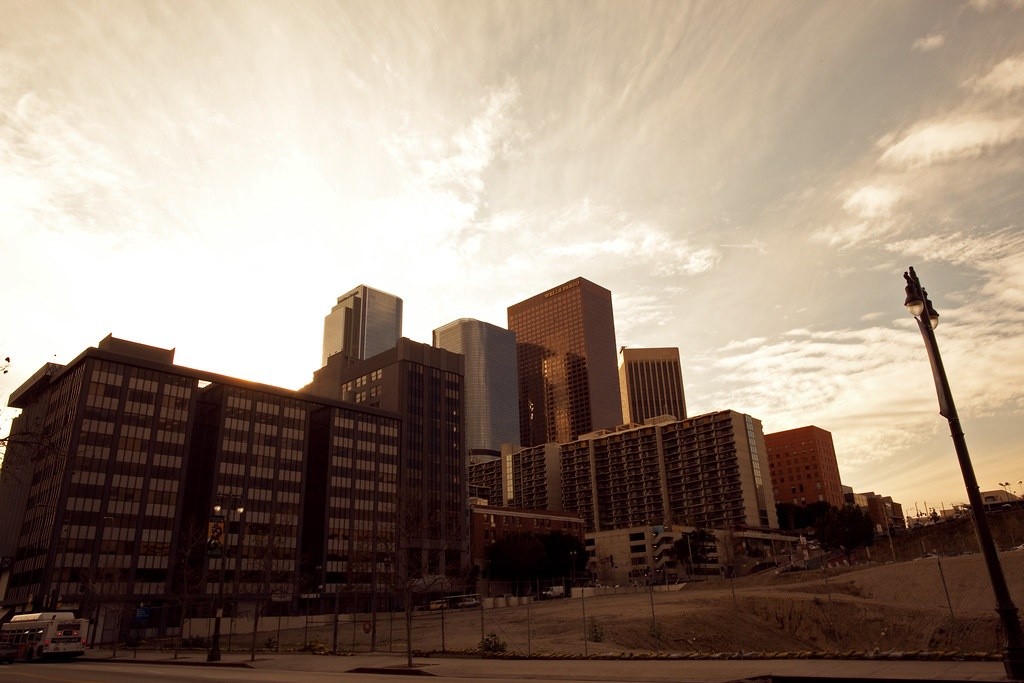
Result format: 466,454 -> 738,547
584,573 -> 683,589
0,642 -> 18,664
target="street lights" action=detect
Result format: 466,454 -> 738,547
206,488 -> 247,663
904,265 -> 1024,680
36,502 -> 71,611
90,517 -> 114,649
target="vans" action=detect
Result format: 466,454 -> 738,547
429,600 -> 448,611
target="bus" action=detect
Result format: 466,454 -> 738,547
443,594 -> 481,609
0,611 -> 86,662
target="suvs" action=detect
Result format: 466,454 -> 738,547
540,586 -> 565,600
